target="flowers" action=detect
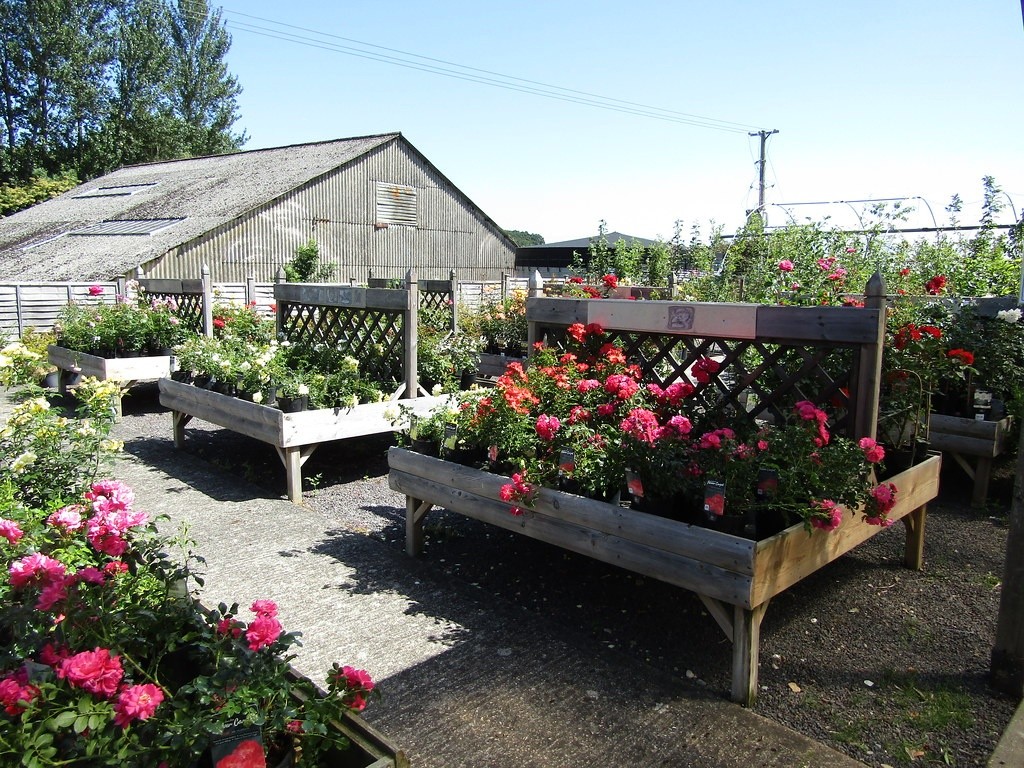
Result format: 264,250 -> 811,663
0,247 -> 1024,768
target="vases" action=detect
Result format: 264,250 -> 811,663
42,370 -> 59,387
218,373 -> 240,393
277,394 -> 308,412
243,384 -> 278,404
58,339 -> 69,348
171,368 -> 193,386
155,344 -> 174,356
120,346 -> 141,357
194,371 -> 215,392
460,371 -> 478,389
93,344 -> 108,357
